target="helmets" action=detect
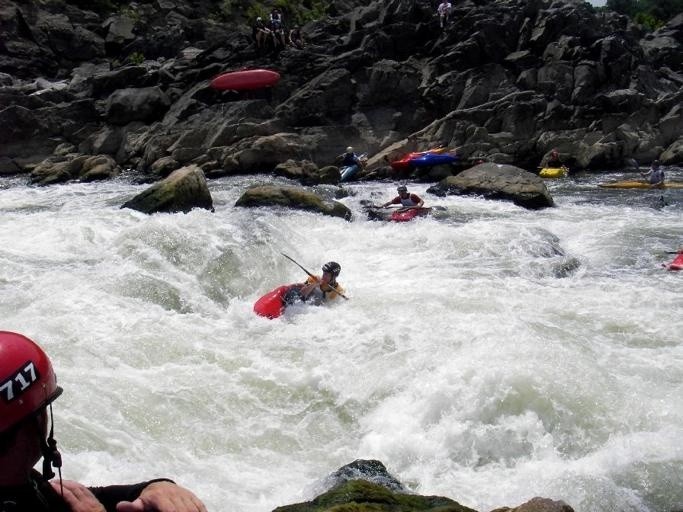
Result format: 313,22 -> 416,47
397,185 -> 407,191
322,262 -> 341,277
0,331 -> 63,435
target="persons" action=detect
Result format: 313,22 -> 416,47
0,331 -> 208,512
381,185 -> 424,210
398,136 -> 424,159
546,151 -> 566,168
252,10 -> 305,63
641,160 -> 664,187
336,147 -> 363,183
677,248 -> 683,254
437,0 -> 452,40
283,262 -> 346,309
2,101 -> 18,134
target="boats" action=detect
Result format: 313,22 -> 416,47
338,152 -> 369,182
538,166 -> 567,178
253,282 -> 344,320
660,250 -> 683,270
389,206 -> 432,222
406,153 -> 459,167
388,144 -> 447,170
209,66 -> 280,89
597,180 -> 683,189
